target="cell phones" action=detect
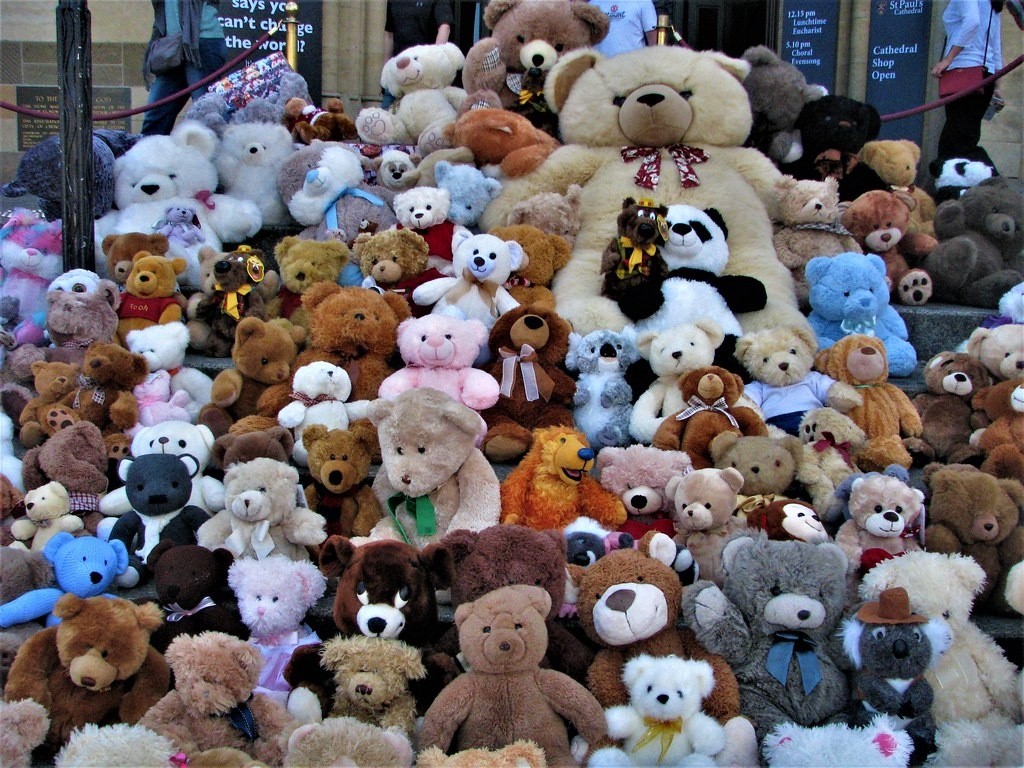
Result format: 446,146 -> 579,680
983,96 -> 1004,121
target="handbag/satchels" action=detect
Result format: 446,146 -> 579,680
938,62 -> 987,99
142,28 -> 184,73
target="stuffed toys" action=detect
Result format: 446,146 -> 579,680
413,230 -> 523,365
278,361 -> 371,467
680,527 -> 857,742
125,320 -> 214,424
378,314 -> 499,445
629,318 -> 787,442
349,386 -> 501,545
837,474 -> 925,603
709,431 -> 804,523
814,333 -> 924,469
2,0 -> 1023,766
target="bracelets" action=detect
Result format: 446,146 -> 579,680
994,90 -> 1000,92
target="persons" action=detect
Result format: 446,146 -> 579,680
931,0 -> 1005,185
140,0 -> 226,135
588,0 -> 659,59
